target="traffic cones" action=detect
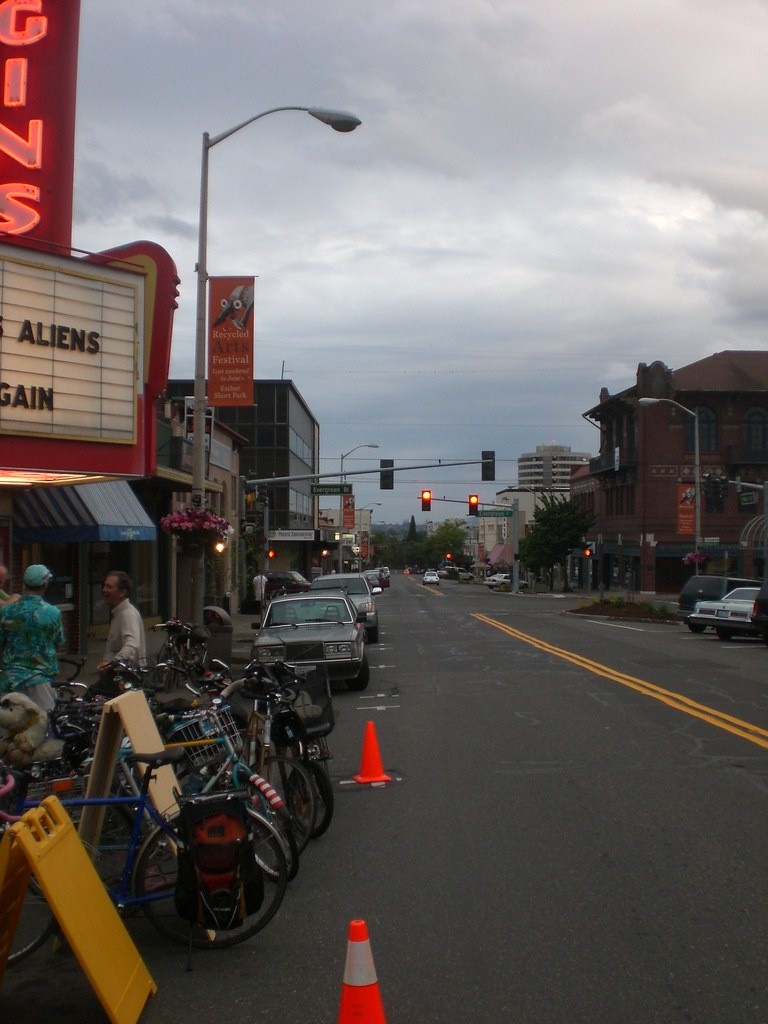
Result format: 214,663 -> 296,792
354,721 -> 392,784
336,921 -> 388,1024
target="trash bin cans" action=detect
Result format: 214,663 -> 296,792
202,606 -> 235,674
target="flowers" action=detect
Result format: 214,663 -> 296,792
682,552 -> 708,572
160,506 -> 234,545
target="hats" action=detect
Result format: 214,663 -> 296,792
25,564 -> 54,586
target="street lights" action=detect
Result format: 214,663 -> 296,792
638,397 -> 701,576
189,106 -> 363,638
339,445 -> 379,573
359,502 -> 384,572
508,485 -> 536,525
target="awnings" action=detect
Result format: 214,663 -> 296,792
13,481 -> 157,543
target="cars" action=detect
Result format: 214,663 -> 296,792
403,566 -> 529,589
251,591 -> 370,691
677,574 -> 768,646
265,571 -> 313,601
308,573 -> 383,644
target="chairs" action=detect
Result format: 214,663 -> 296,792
324,605 -> 341,620
281,607 -> 298,620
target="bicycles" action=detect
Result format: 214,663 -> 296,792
0,618 -> 337,971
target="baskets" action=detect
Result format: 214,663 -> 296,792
175,707 -> 245,765
292,697 -> 336,739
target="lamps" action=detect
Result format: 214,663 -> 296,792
153,388 -> 174,419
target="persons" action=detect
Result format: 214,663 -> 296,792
96,571 -> 146,687
0,564 -> 66,711
253,572 -> 268,601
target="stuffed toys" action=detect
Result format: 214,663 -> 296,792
0,692 -> 66,767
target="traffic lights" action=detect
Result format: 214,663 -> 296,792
256,484 -> 268,502
266,549 -> 277,560
469,495 -> 478,515
585,549 -> 593,557
422,491 -> 431,511
719,476 -> 728,498
702,474 -> 711,494
